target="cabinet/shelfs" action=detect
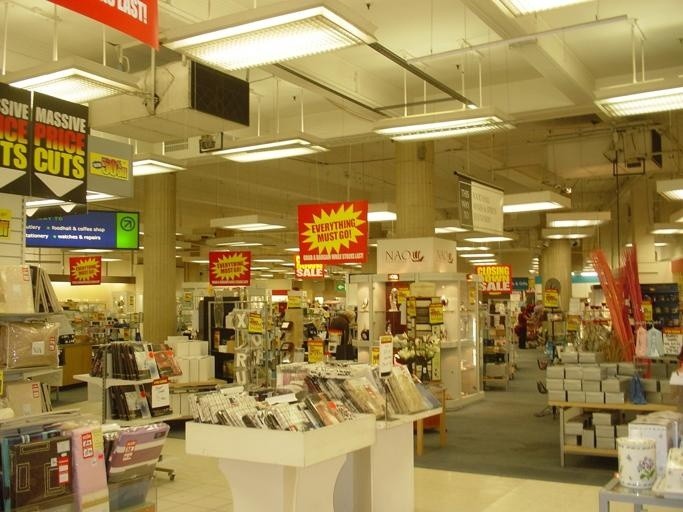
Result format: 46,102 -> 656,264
0,273 -> 683,512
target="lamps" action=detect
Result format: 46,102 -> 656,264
210,77 -> 332,164
650,110 -> 683,248
0,0 -> 139,104
159,0 -> 378,74
127,136 -> 188,176
435,129 -> 611,265
177,156 -> 299,272
492,0 -> 591,19
592,18 -> 683,119
371,39 -> 517,143
347,141 -> 398,223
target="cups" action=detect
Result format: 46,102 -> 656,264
614,436 -> 657,491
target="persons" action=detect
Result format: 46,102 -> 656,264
518,306 -> 534,349
